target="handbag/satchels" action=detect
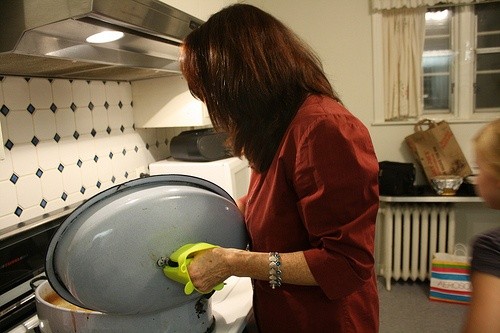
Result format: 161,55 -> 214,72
429,243 -> 472,305
405,118 -> 473,188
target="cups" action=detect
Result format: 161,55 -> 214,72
431,176 -> 463,196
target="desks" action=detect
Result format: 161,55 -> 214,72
379,196 -> 488,202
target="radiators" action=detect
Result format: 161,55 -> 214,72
379,203 -> 456,291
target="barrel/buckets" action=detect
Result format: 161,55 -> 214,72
377,161 -> 415,197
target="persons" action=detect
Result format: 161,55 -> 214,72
463,118 -> 500,333
178,3 -> 381,333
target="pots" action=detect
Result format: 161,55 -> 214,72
30,175 -> 252,333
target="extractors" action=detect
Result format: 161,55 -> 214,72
0,1 -> 207,82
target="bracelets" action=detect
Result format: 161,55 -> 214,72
268,252 -> 282,290
